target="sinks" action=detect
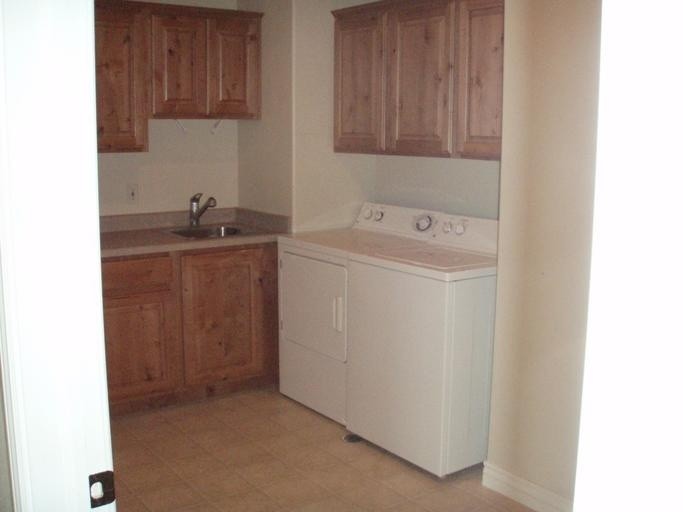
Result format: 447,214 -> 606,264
167,224 -> 241,240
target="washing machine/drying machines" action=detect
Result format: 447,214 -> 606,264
345,212 -> 498,478
276,205 -> 415,428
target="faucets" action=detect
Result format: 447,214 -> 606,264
190,193 -> 216,227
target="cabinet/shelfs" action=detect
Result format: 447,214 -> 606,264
99,241 -> 278,420
330,1 -> 504,162
151,1 -> 262,120
95,1 -> 149,153
280,250 -> 348,364
